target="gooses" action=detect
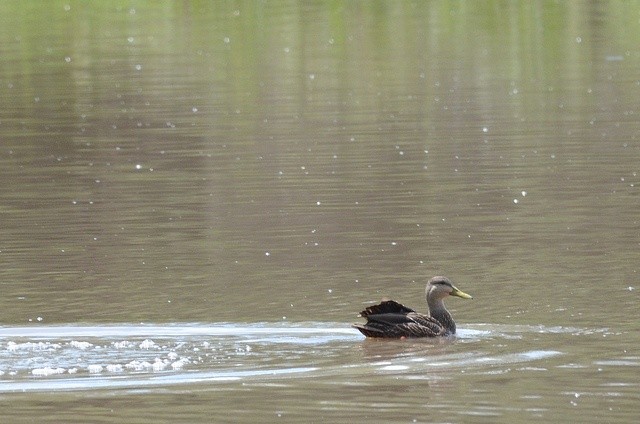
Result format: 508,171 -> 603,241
351,274 -> 471,339
